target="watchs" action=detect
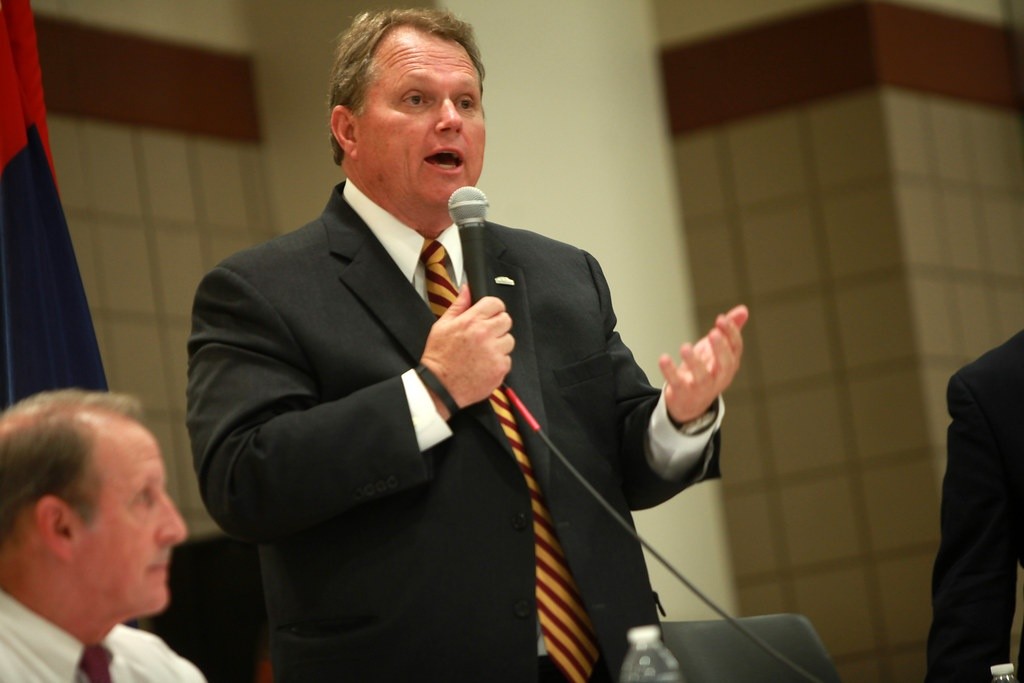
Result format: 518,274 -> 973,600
667,401 -> 716,435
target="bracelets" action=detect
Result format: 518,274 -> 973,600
417,367 -> 460,416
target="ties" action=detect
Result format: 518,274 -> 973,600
420,238 -> 601,683
80,646 -> 111,683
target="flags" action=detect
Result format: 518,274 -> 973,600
0,0 -> 107,412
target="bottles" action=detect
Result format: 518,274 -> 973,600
991,663 -> 1018,683
621,625 -> 681,683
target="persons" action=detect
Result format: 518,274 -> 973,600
186,9 -> 749,683
925,329 -> 1024,683
0,389 -> 205,683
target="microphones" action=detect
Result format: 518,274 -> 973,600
449,186 -> 494,307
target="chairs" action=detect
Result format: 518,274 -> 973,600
659,611 -> 842,682
